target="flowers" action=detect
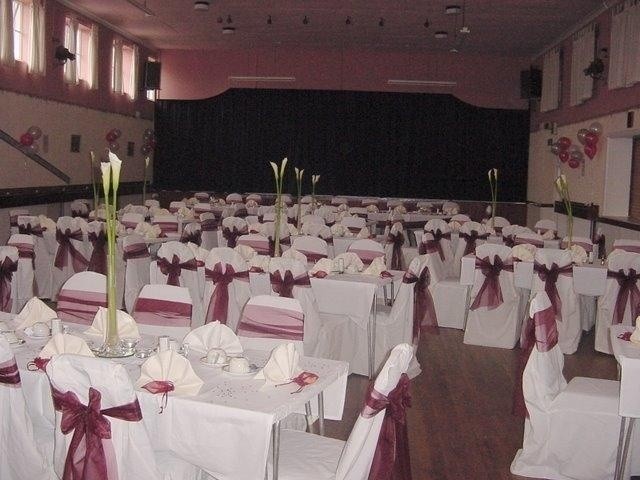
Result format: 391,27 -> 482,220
552,172 -> 576,252
98,151 -> 129,334
310,172 -> 320,202
486,164 -> 498,212
269,156 -> 288,223
292,167 -> 307,208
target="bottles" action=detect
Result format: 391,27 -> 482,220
338,258 -> 344,274
600,254 -> 605,266
588,251 -> 594,265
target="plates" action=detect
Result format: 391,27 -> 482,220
12,338 -> 24,349
221,364 -> 258,376
199,355 -> 228,367
153,348 -> 185,356
32,331 -> 65,339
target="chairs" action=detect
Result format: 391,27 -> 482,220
511,291 -> 640,477
0,192 -> 443,479
421,213 -> 640,352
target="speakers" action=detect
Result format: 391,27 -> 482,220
521,68 -> 542,99
142,61 -> 161,89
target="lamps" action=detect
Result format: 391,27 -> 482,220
217,15 -> 434,30
386,48 -> 457,88
222,26 -> 236,35
443,3 -> 464,16
227,44 -> 297,83
433,31 -> 450,39
192,0 -> 212,13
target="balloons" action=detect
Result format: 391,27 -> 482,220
106,133 -> 115,141
585,132 -> 599,147
109,140 -> 119,151
29,142 -> 41,155
561,138 -> 571,150
560,151 -> 569,162
27,126 -> 42,140
112,128 -> 121,138
570,151 -> 582,160
577,128 -> 590,145
589,122 -> 603,137
141,127 -> 157,154
551,142 -> 561,155
21,134 -> 33,146
567,158 -> 579,168
584,144 -> 597,159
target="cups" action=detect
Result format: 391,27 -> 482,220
158,336 -> 181,352
229,357 -> 250,374
25,322 -> 51,336
1,330 -> 17,343
0,321 -> 9,330
207,347 -> 228,364
51,318 -> 63,335
